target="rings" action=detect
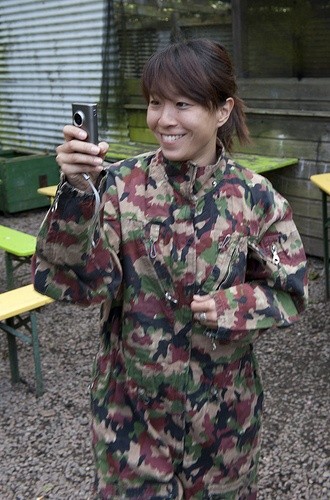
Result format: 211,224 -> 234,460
200,313 -> 206,322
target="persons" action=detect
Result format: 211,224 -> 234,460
32,39 -> 309,500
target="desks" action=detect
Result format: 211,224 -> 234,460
0,225 -> 38,290
91,144 -> 298,174
311,172 -> 330,299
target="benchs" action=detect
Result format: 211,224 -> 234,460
37,185 -> 59,206
0,283 -> 57,397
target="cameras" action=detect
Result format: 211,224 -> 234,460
72,102 -> 99,157
